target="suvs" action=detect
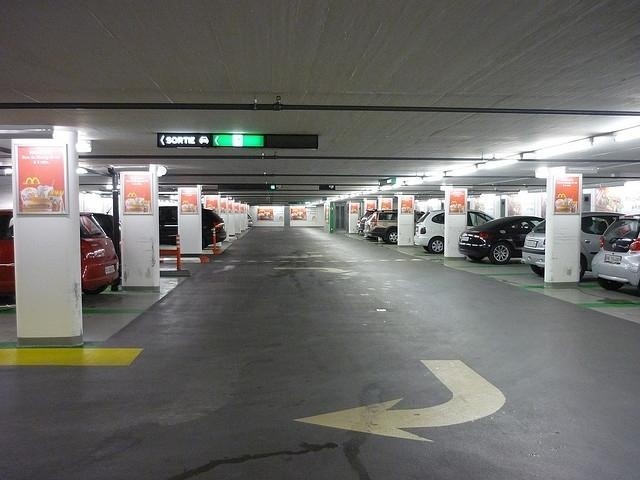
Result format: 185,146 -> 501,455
160,206 -> 225,248
357,208 -> 422,243
0,210 -> 119,306
414,210 -> 494,252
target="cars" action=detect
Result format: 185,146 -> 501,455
458,216 -> 544,263
522,212 -> 640,289
